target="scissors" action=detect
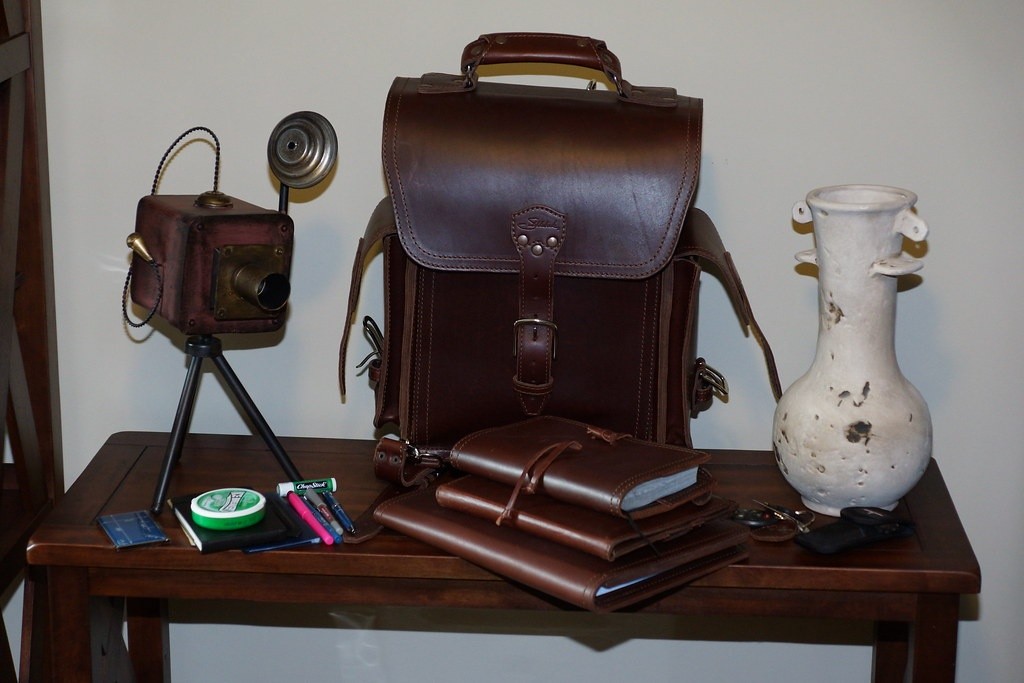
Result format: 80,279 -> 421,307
750,498 -> 818,534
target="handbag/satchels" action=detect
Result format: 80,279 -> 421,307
339,32 -> 784,488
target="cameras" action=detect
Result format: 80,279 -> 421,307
130,189 -> 294,338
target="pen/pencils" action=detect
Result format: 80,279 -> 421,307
305,489 -> 345,535
324,490 -> 358,535
304,496 -> 343,544
246,537 -> 321,554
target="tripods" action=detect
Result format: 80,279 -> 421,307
149,338 -> 304,518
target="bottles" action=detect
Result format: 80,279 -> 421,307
771,181 -> 934,519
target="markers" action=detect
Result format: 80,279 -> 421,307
287,491 -> 334,545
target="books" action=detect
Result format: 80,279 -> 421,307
373,414 -> 754,614
166,487 -> 323,555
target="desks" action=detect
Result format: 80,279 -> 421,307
22,432 -> 983,682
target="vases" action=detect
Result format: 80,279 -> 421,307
771,185 -> 933,518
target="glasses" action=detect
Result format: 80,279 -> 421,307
730,497 -> 817,542
